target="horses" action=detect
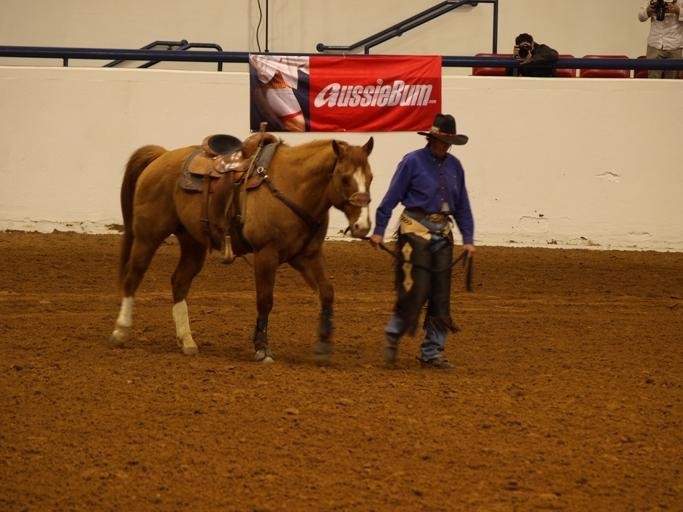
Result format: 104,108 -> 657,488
108,133 -> 375,365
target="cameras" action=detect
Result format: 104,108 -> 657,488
518,43 -> 532,58
650,0 -> 668,21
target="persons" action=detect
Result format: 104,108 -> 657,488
638,0 -> 683,78
513,34 -> 560,77
368,111 -> 475,373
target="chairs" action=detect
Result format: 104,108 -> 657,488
472,53 -> 683,79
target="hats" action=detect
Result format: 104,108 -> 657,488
417,114 -> 468,145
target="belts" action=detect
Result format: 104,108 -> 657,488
411,209 -> 449,223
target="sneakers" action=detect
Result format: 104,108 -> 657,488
386,346 -> 397,363
431,359 -> 453,369
